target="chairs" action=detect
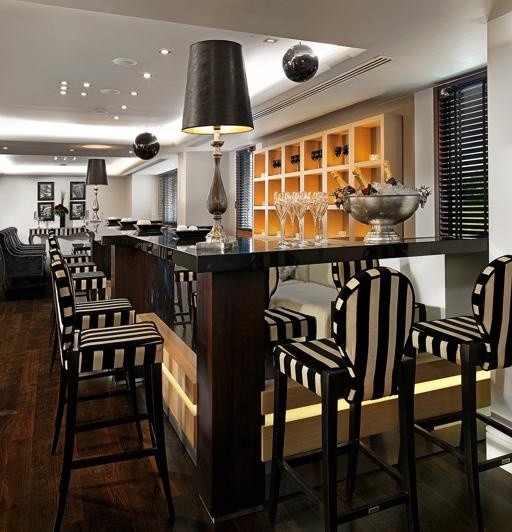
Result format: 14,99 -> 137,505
1,226 -> 46,291
264,265 -> 317,378
172,265 -> 198,355
48,227 -> 177,531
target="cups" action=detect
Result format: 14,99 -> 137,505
160,225 -> 180,241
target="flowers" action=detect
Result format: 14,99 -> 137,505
54,204 -> 69,217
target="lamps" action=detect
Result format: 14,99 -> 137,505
84,158 -> 110,223
181,39 -> 254,251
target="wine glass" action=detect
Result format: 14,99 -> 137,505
311,149 -> 323,169
291,155 -> 300,172
272,160 -> 281,174
273,191 -> 329,248
335,194 -> 426,243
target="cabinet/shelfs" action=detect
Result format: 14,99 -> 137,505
250,112 -> 403,240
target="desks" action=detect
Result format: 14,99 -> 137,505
29,226 -> 89,244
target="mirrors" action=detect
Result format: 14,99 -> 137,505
264,254 -> 447,390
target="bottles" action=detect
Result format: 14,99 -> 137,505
331,169 -> 356,195
351,166 -> 378,196
382,160 -> 398,186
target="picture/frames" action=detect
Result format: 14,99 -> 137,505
69,181 -> 86,221
36,180 -> 56,221
